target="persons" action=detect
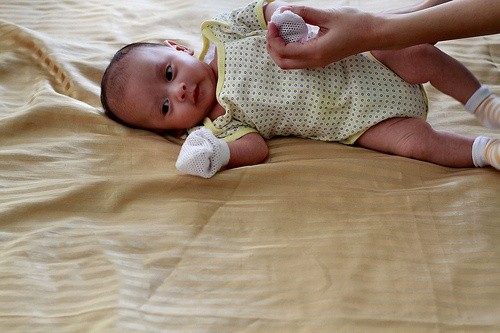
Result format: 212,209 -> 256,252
266,0 -> 500,70
100,0 -> 500,179
